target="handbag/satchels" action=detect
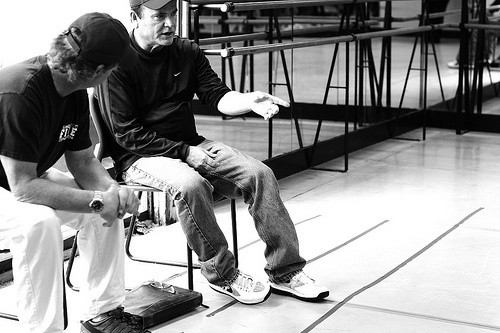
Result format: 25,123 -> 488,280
118,279 -> 209,328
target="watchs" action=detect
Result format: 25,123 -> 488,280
88,191 -> 104,214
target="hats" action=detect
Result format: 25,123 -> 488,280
64,13 -> 139,69
129,1 -> 191,11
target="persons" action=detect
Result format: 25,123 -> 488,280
0,12 -> 151,333
447,0 -> 500,71
93,0 -> 329,305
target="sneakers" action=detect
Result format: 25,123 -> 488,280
79,305 -> 153,333
269,270 -> 329,302
208,271 -> 271,305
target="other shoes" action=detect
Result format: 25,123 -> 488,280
448,56 -> 499,71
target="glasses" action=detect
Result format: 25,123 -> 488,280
150,280 -> 176,295
